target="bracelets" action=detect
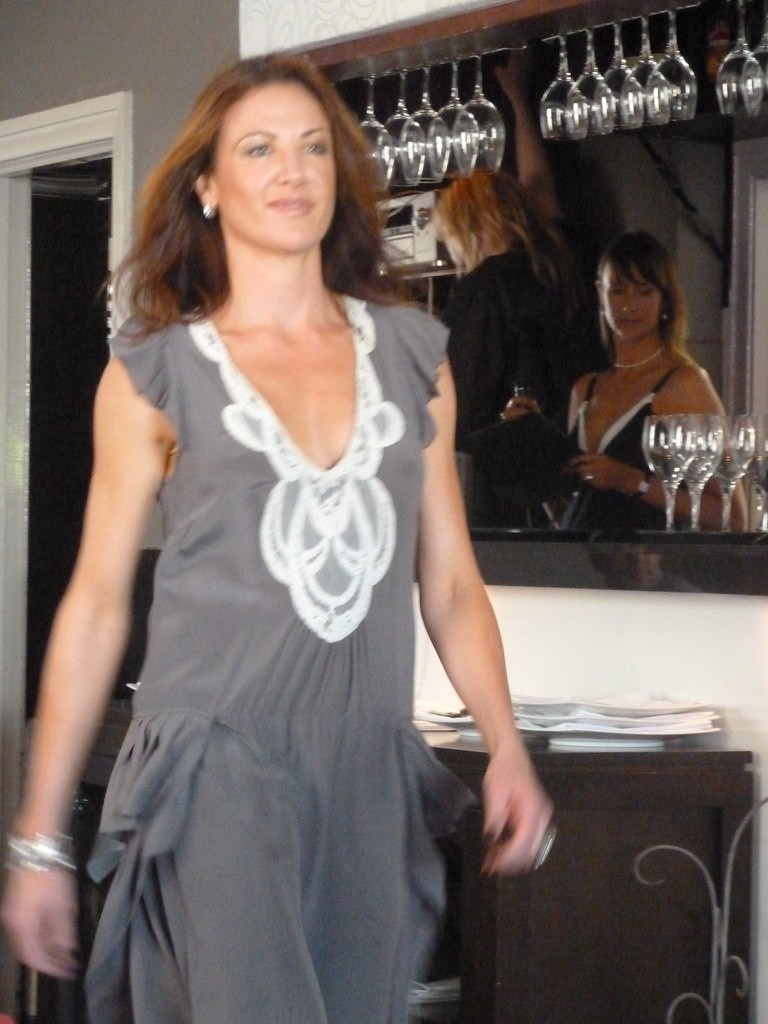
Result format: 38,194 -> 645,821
8,837 -> 73,872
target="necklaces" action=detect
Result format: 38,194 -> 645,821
615,346 -> 665,368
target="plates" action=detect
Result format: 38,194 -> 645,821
414,693 -> 722,748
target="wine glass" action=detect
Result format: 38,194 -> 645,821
367,53 -> 503,188
642,414 -> 767,534
717,1 -> 768,117
540,7 -> 699,140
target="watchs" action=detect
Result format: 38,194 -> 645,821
634,470 -> 651,497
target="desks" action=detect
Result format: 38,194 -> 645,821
50,706 -> 756,1024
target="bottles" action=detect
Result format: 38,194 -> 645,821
514,383 -> 530,398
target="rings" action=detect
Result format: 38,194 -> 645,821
584,475 -> 593,481
544,833 -> 554,846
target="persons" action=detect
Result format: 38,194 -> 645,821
499,231 -> 748,536
431,173 -> 603,530
2,37 -> 560,1024
